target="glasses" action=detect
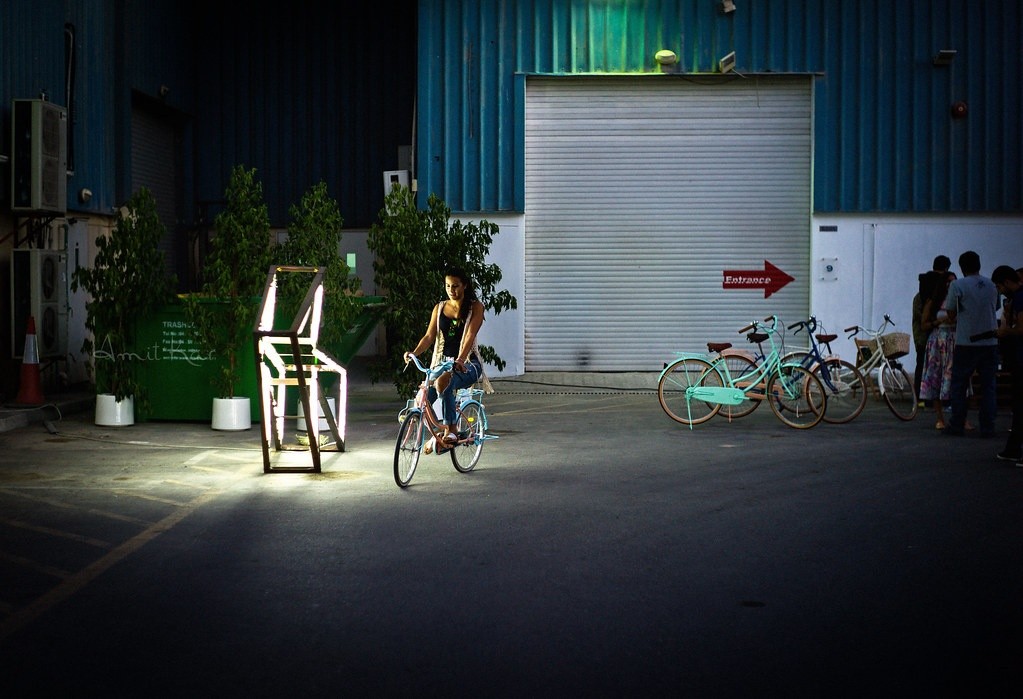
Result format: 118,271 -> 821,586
447,317 -> 463,338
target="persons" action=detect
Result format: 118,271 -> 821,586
913,255 -> 951,410
920,272 -> 976,430
947,251 -> 1001,430
404,267 -> 485,455
991,264 -> 1022,467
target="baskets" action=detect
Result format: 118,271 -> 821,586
868,332 -> 910,359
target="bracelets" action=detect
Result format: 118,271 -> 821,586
458,357 -> 465,363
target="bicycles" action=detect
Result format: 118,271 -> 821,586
391,350 -> 499,489
654,316 -> 826,429
701,315 -> 868,424
769,311 -> 919,420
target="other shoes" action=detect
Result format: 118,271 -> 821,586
996,448 -> 1023,468
423,432 -> 440,455
442,432 -> 459,443
915,394 -> 997,437
